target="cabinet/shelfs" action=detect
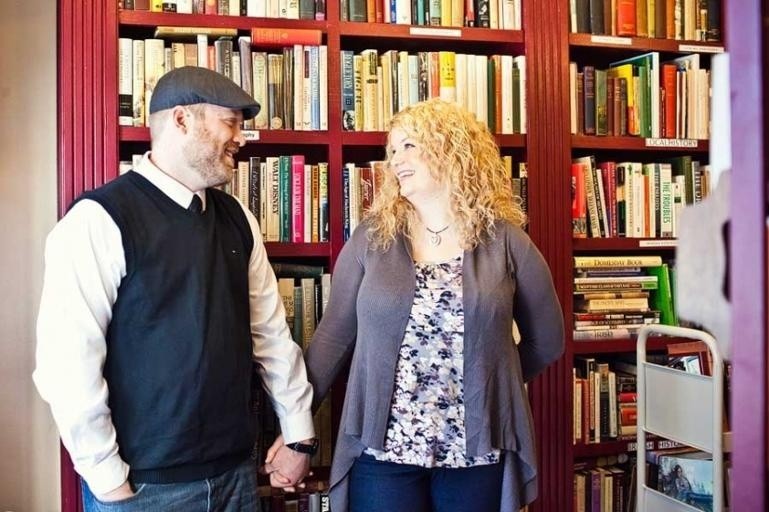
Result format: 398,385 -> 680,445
50,0 -> 731,512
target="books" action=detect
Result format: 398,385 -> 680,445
571,358 -> 733,511
310,409 -> 320,467
257,480 -> 331,511
320,389 -> 333,466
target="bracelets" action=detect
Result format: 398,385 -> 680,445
287,438 -> 319,457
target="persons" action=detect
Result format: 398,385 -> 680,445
30,65 -> 319,511
264,97 -> 565,511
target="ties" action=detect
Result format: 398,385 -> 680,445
188,194 -> 204,215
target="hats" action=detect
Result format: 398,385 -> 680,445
149,65 -> 262,122
